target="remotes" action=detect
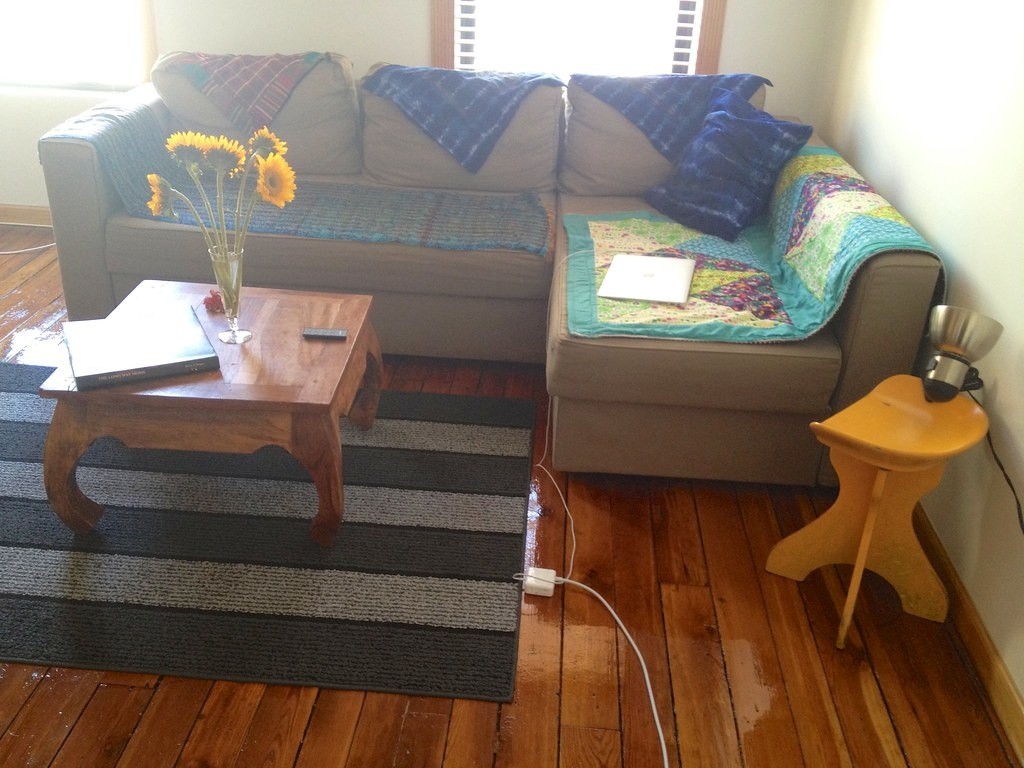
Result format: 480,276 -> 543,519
301,328 -> 348,340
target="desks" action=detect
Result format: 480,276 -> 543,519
765,373 -> 990,649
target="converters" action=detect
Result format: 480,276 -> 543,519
525,567 -> 557,596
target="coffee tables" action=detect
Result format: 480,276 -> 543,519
38,279 -> 384,546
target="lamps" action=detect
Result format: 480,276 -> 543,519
923,304 -> 1006,402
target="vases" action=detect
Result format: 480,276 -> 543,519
208,244 -> 252,344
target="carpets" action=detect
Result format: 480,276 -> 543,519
0,361 -> 537,701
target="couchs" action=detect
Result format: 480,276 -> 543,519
37,49 -> 947,488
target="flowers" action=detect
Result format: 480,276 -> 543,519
203,289 -> 225,313
146,124 -> 297,314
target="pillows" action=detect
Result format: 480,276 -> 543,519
642,84 -> 813,243
